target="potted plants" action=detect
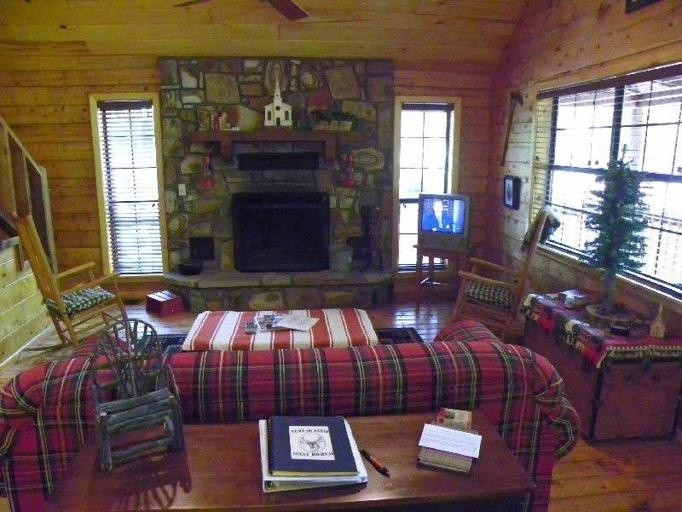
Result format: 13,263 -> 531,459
577,145 -> 650,328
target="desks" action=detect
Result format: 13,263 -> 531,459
413,231 -> 496,315
50,408 -> 536,507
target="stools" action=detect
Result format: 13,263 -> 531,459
144,288 -> 183,317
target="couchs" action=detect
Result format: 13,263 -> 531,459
0,319 -> 581,510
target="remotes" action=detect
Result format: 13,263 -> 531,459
245,321 -> 255,333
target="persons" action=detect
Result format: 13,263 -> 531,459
422,198 -> 453,233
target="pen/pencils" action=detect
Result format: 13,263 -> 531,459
358,448 -> 391,477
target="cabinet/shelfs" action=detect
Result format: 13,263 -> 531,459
523,286 -> 681,449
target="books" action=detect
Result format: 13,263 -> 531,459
266,415 -> 359,477
416,420 -> 479,475
559,287 -> 589,307
277,312 -> 319,331
252,314 -> 289,332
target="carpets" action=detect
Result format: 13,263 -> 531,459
374,327 -> 425,347
138,335 -> 187,353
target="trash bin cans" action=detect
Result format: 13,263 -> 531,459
328,244 -> 354,273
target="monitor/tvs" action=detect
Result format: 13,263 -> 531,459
417,193 -> 471,248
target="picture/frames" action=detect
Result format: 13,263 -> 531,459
501,174 -> 521,210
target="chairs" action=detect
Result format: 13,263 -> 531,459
8,210 -> 135,348
446,207 -> 553,338
87,319 -> 185,474
